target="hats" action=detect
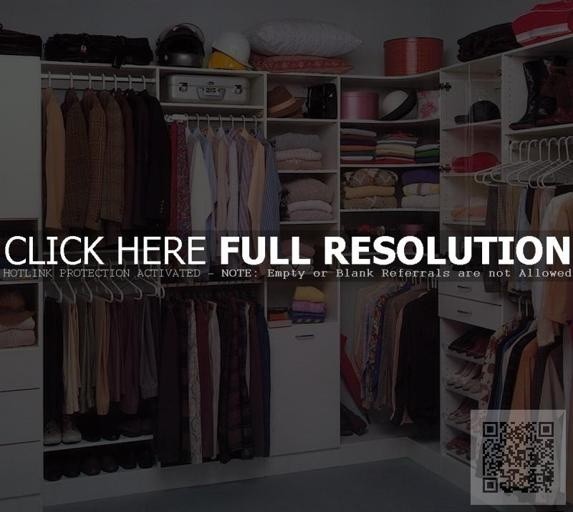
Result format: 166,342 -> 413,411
454,152 -> 496,173
381,90 -> 416,121
267,87 -> 303,117
455,101 -> 502,122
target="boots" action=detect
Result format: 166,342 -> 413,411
508,56 -> 573,129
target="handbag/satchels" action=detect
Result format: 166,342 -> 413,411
308,81 -> 338,120
512,1 -> 573,47
457,22 -> 522,62
43,33 -> 153,68
0,24 -> 42,57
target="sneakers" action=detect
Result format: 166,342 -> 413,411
43,441 -> 152,482
44,408 -> 156,444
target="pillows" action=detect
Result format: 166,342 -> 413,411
246,19 -> 364,56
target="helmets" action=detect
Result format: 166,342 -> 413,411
158,23 -> 205,67
209,32 -> 255,70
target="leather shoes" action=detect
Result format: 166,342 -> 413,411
446,331 -> 490,461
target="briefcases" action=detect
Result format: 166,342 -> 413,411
164,73 -> 249,104
